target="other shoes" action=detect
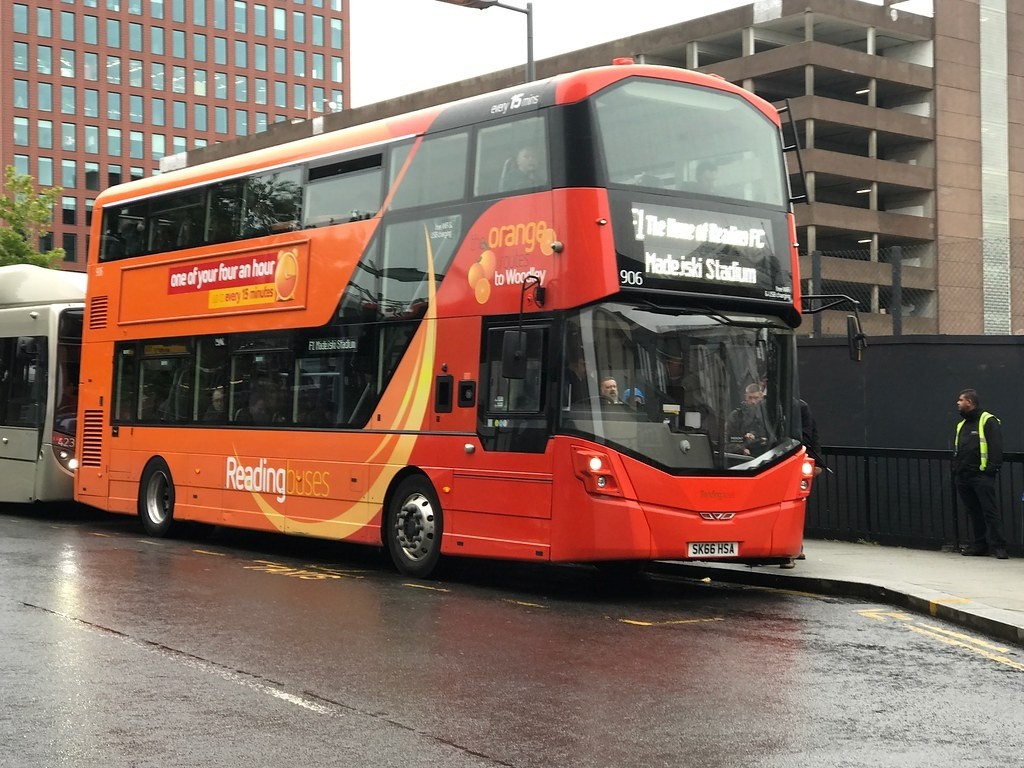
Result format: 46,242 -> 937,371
798,545 -> 805,559
962,548 -> 989,556
996,548 -> 1008,559
780,556 -> 795,568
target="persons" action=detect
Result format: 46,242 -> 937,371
599,372 -> 647,411
496,136 -> 732,198
134,356 -> 392,431
557,352 -> 610,407
952,388 -> 1009,560
651,349 -> 709,408
726,381 -> 823,570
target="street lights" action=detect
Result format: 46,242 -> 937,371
441,0 -> 535,86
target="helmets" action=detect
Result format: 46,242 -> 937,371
623,388 -> 645,402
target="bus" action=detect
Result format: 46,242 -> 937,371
0,263 -> 87,510
72,56 -> 869,587
